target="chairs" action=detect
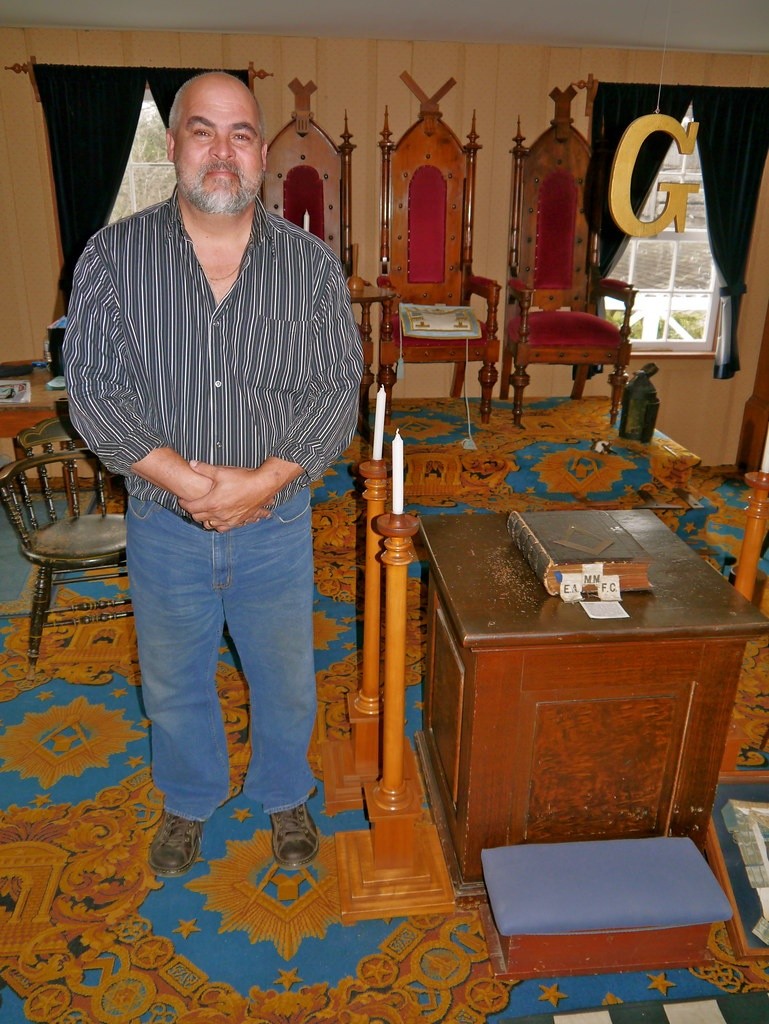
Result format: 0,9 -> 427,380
499,113 -> 639,426
0,447 -> 135,666
261,108 -> 375,443
374,104 -> 504,425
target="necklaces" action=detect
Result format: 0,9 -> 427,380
208,265 -> 239,280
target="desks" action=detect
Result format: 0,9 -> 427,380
411,507 -> 769,905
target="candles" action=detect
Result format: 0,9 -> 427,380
391,427 -> 405,514
372,383 -> 387,459
761,424 -> 769,472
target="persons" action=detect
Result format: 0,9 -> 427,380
61,71 -> 363,878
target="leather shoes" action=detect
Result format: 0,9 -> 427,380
270,802 -> 320,870
147,811 -> 202,877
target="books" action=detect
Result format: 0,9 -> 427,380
507,510 -> 654,597
